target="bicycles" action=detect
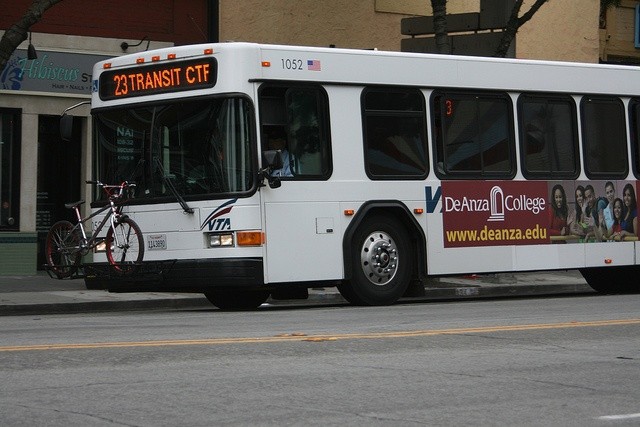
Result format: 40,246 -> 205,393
45,180 -> 144,277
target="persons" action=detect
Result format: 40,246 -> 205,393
609,183 -> 638,241
584,181 -> 615,239
572,185 -> 609,243
599,198 -> 624,241
570,184 -> 593,243
548,184 -> 576,244
263,135 -> 301,178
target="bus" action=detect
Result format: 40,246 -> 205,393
58,41 -> 640,310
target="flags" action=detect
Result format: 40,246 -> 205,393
307,60 -> 321,72
